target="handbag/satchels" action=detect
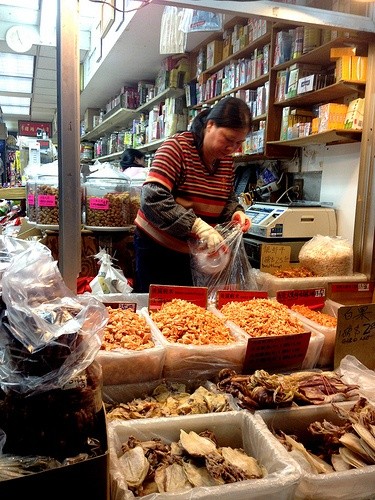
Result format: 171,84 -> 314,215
191,223 -> 259,292
89,254 -> 134,295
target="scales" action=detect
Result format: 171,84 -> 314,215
244,200 -> 338,240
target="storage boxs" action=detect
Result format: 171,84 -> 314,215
330,47 -> 368,82
274,63 -> 322,102
84,56 -> 190,132
274,27 -> 304,65
280,98 -> 366,142
0,299 -> 375,500
188,19 -> 271,129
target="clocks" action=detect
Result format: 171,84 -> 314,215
5,24 -> 32,53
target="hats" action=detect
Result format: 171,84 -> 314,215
11,205 -> 20,211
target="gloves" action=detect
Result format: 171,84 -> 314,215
190,217 -> 230,260
232,211 -> 251,234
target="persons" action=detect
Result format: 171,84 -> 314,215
132,96 -> 254,292
119,148 -> 152,184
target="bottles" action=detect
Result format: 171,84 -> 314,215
26,173 -> 145,228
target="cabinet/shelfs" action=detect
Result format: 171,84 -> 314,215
80,108 -> 136,162
188,17 -> 292,161
133,88 -> 186,150
266,0 -> 369,146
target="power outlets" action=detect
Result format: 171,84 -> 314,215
293,178 -> 303,199
